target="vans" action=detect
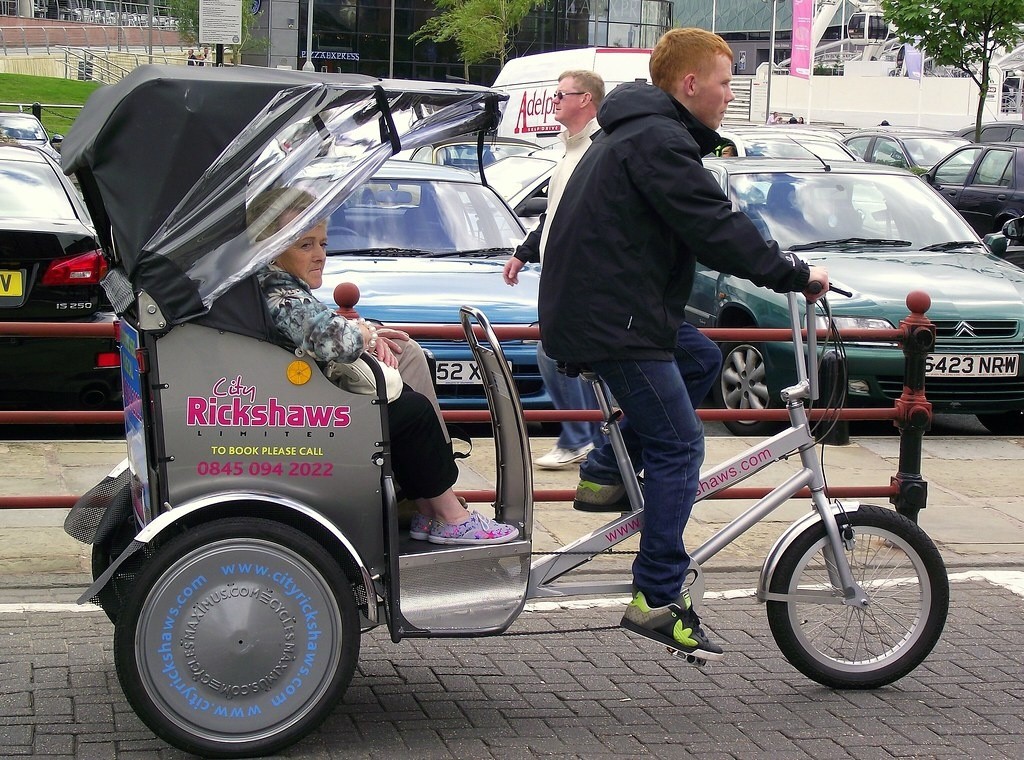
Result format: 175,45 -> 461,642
490,48 -> 655,149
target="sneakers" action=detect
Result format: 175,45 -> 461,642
620,590 -> 725,662
533,439 -> 596,468
428,509 -> 520,545
409,511 -> 436,540
574,476 -> 646,513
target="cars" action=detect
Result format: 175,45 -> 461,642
0,112 -> 124,442
243,105 -> 1024,439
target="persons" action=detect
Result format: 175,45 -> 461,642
247,188 -> 519,544
881,120 -> 889,126
503,69 -> 627,468
768,112 -> 804,124
187,48 -> 212,67
539,28 -> 830,663
718,143 -> 766,182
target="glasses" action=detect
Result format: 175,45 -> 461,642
554,92 -> 592,101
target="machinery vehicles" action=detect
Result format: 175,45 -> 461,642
811,0 -> 907,77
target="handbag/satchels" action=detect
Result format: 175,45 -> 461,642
323,347 -> 403,405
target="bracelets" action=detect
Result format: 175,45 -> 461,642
352,318 -> 378,351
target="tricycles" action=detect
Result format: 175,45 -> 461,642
56,65 -> 949,760
